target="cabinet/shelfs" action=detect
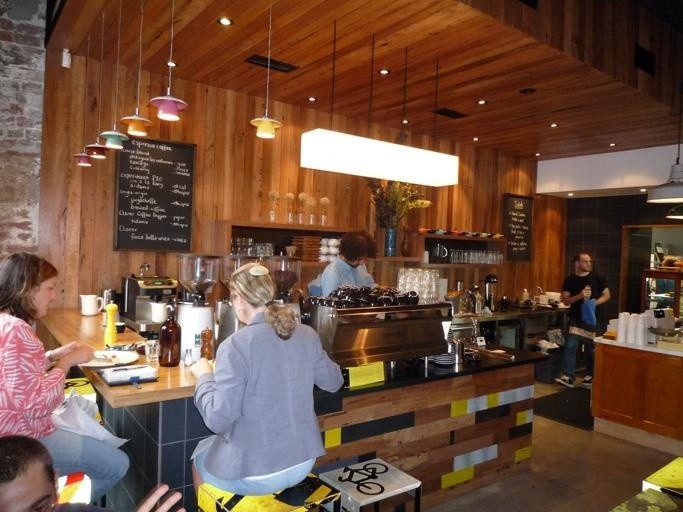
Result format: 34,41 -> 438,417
591,338 -> 683,457
412,234 -> 507,270
217,220 -> 354,264
476,309 -> 571,352
642,270 -> 683,323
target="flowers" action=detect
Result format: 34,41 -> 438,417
363,174 -> 431,229
299,193 -> 308,208
284,193 -> 295,215
306,197 -> 316,210
319,196 -> 330,212
444,286 -> 480,314
268,191 -> 279,204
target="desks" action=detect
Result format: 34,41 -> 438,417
41,306 -> 199,410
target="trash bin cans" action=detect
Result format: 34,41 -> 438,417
533,343 -> 565,384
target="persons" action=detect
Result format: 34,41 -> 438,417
190,263 -> 345,498
1,435 -> 188,512
321,228 -> 379,298
554,252 -> 611,389
0,251 -> 131,505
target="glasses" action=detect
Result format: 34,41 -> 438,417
38,482 -> 58,512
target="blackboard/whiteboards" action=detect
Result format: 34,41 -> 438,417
113,134 -> 197,253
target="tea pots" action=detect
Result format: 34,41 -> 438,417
320,238 -> 341,263
432,242 -> 448,258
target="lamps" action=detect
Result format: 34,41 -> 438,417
71,30 -> 92,167
99,0 -> 129,149
85,13 -> 109,160
248,2 -> 284,140
299,18 -> 459,187
645,114 -> 683,202
121,0 -> 153,137
665,205 -> 683,220
149,0 -> 189,124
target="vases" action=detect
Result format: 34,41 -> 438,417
320,209 -> 327,228
270,207 -> 278,223
384,228 -> 396,258
298,207 -> 305,225
288,208 -> 295,225
452,312 -> 475,343
309,208 -> 315,225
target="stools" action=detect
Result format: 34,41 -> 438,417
319,457 -> 423,511
197,471 -> 341,512
53,472 -> 92,506
53,377 -> 102,507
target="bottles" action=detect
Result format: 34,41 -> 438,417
418,227 -> 507,239
519,288 -> 530,307
103,300 -> 118,344
159,307 -> 213,366
500,293 -> 508,312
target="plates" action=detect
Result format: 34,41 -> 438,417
290,235 -> 322,261
421,329 -> 454,365
77,350 -> 140,367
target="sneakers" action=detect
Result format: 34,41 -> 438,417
581,375 -> 593,386
554,374 -> 576,389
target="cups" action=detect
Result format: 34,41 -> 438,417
79,287 -> 117,315
617,312 -> 648,345
145,339 -> 158,363
396,268 -> 440,305
115,323 -> 125,333
306,285 -> 419,308
148,302 -> 174,325
230,236 -> 274,257
450,248 -> 505,265
539,295 -> 550,304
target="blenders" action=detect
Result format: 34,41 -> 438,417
180,252 -> 302,362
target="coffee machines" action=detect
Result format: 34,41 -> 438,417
121,263 -> 179,339
